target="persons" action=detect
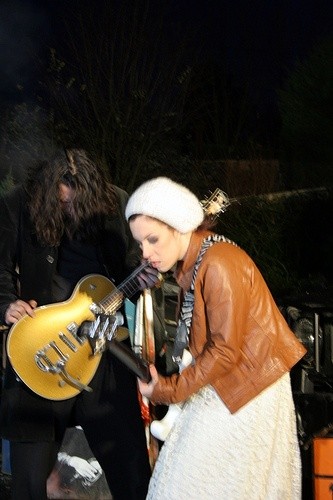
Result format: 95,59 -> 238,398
0,149 -> 168,500
125,177 -> 307,500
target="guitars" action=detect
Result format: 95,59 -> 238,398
6,187 -> 232,403
78,312 -> 194,441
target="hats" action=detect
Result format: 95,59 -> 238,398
125,175 -> 205,233
